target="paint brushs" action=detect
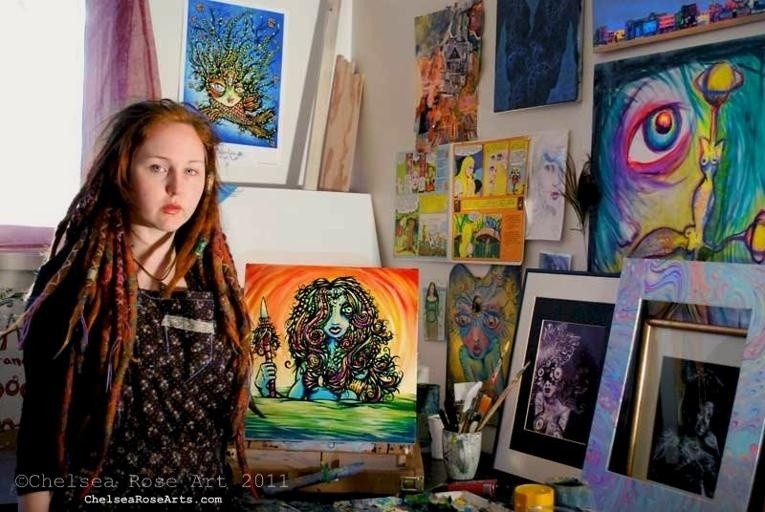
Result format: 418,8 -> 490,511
476,340 -> 531,433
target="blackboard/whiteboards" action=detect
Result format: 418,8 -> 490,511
217,184 -> 383,288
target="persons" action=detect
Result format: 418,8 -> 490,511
645,364 -> 726,498
2,96 -> 269,512
531,364 -> 572,439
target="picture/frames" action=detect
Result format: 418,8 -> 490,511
592,0 -> 765,54
627,318 -> 748,499
494,268 -> 621,483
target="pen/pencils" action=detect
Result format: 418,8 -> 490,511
437,390 -> 498,433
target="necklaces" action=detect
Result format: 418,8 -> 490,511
126,245 -> 182,292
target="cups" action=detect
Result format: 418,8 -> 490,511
440,427 -> 482,481
542,475 -> 593,511
513,482 -> 555,512
426,413 -> 455,460
